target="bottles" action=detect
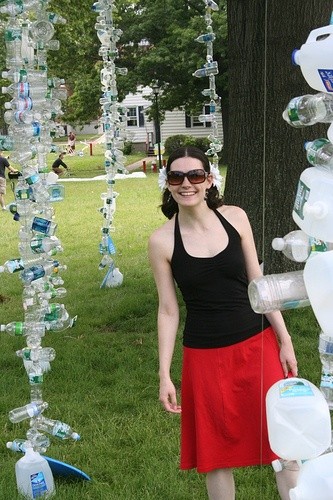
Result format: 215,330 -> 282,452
192,67 -> 218,78
92,0 -> 129,272
0,1 -> 83,457
15,448 -> 56,500
194,33 -> 216,43
198,89 -> 222,180
247,23 -> 333,500
203,61 -> 218,68
202,0 -> 219,10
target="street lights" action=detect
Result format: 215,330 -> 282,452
151,82 -> 162,168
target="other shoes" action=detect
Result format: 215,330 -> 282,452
2,205 -> 6,210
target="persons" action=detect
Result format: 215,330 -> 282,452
68,131 -> 76,151
148,144 -> 302,500
0,151 -> 18,210
52,153 -> 70,176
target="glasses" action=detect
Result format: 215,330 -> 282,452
165,169 -> 210,186
